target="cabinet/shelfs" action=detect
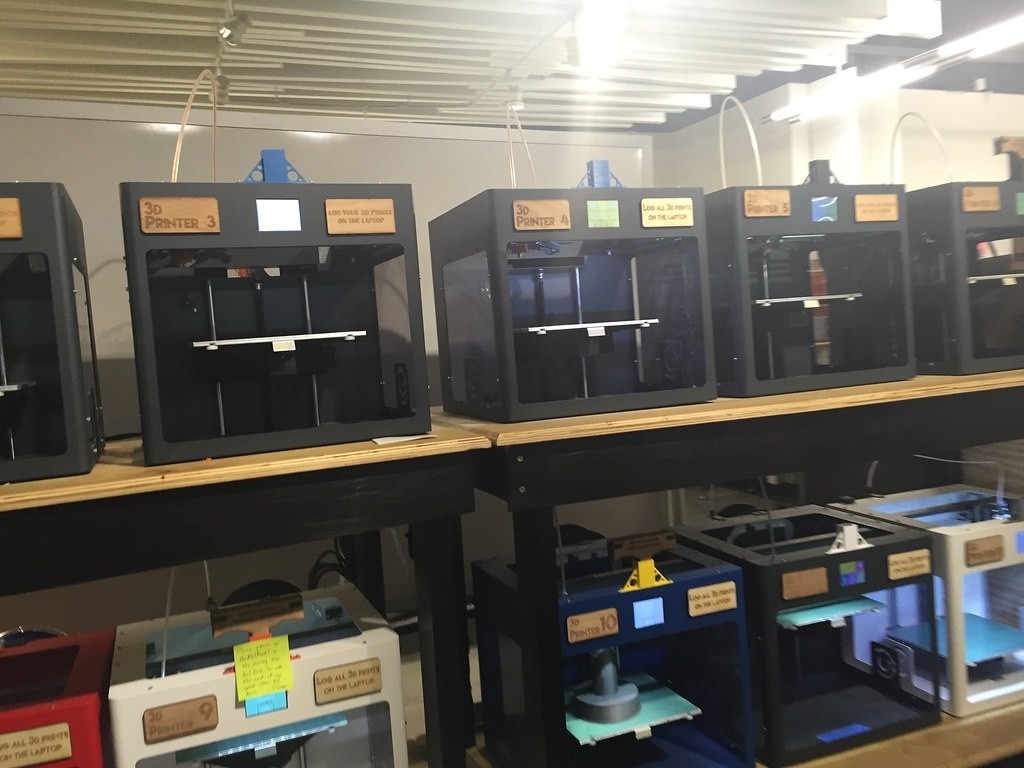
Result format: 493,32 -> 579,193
0,424 -> 492,768
430,370 -> 1024,768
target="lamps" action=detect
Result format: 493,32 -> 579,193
216,0 -> 252,48
503,71 -> 524,110
